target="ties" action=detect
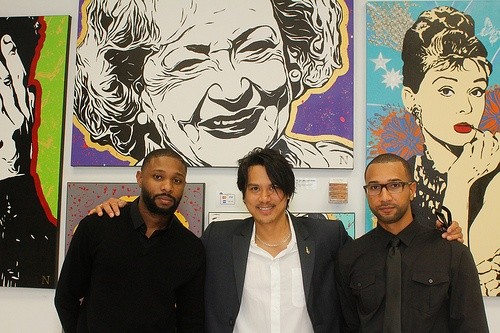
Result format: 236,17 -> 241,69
384,236 -> 402,333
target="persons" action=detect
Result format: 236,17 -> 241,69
89,147 -> 464,333
333,152 -> 490,333
54,148 -> 206,333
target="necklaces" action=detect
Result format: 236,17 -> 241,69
255,231 -> 291,247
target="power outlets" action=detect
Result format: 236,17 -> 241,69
221,195 -> 235,206
294,178 -> 317,189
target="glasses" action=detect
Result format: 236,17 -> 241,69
363,182 -> 411,195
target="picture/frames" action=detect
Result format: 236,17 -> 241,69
208,211 -> 355,241
64,182 -> 205,255
